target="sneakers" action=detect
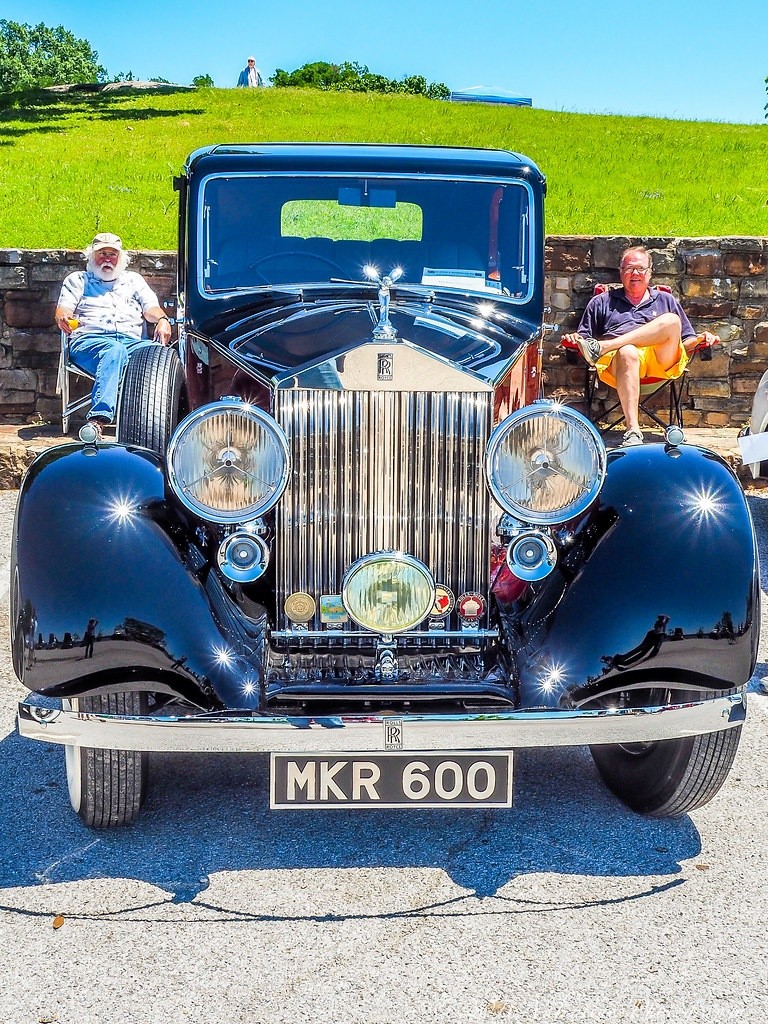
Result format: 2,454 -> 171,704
622,428 -> 644,448
577,338 -> 602,368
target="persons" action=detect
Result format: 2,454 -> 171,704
55,233 -> 171,431
561,246 -> 720,447
237,57 -> 263,87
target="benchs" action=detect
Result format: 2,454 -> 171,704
216,234 -> 501,287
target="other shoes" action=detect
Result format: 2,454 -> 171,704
86,417 -> 104,442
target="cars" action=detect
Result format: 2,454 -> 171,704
738,321 -> 768,480
8,143 -> 766,830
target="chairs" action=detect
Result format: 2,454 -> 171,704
561,283 -> 720,436
56,319 -> 151,443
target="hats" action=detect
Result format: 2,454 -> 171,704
91,232 -> 122,252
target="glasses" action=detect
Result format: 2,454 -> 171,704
99,251 -> 117,258
625,266 -> 650,274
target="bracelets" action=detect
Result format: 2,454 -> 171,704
159,316 -> 168,321
